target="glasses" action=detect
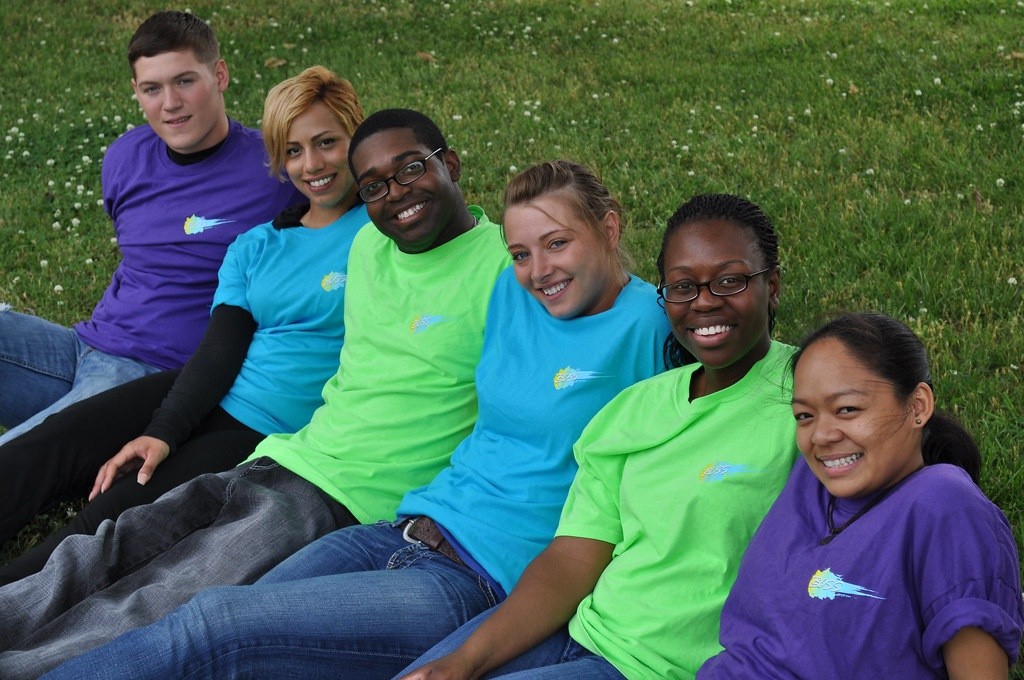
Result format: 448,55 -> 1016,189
656,267 -> 774,303
355,148 -> 443,203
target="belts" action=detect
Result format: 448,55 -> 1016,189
398,515 -> 468,567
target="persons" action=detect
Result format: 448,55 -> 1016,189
38,161 -> 678,680
695,311 -> 1010,680
0,9 -> 514,680
392,192 -> 804,680
